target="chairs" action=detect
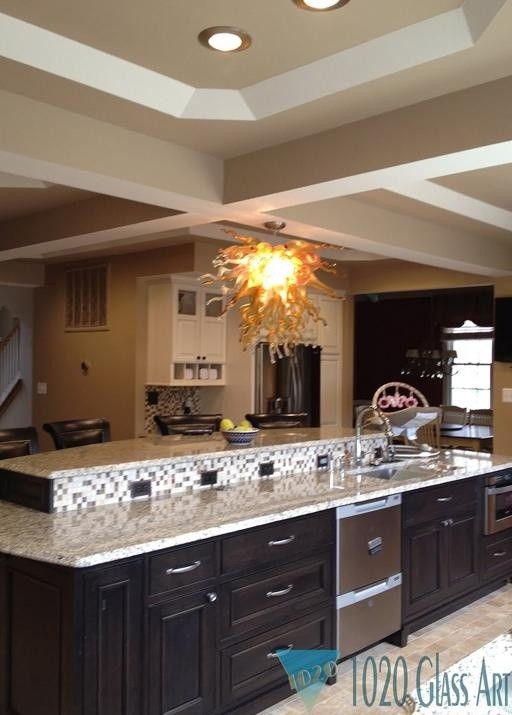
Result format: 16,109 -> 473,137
245,412 -> 310,429
154,414 -> 224,437
0,415 -> 111,458
440,405 -> 493,426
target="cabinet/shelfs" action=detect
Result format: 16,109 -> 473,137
0,476 -> 512,715
139,273 -> 233,391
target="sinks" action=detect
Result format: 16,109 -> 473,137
346,462 -> 438,480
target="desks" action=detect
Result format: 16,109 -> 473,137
440,423 -> 493,454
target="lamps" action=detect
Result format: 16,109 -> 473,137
198,220 -> 347,364
400,296 -> 457,382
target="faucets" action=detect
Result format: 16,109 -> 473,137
354,406 -> 395,466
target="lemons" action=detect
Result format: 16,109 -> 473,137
220,418 -> 251,434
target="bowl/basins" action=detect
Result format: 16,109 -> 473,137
220,427 -> 259,446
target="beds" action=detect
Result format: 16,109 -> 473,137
357,382 -> 442,449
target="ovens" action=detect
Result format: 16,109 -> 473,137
483,473 -> 512,537
